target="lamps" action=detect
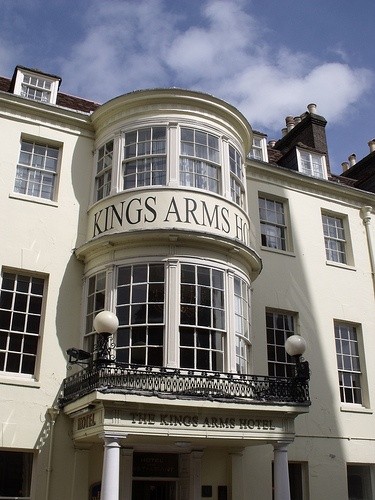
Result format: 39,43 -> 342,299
175,442 -> 192,448
93,311 -> 118,334
284,334 -> 306,357
